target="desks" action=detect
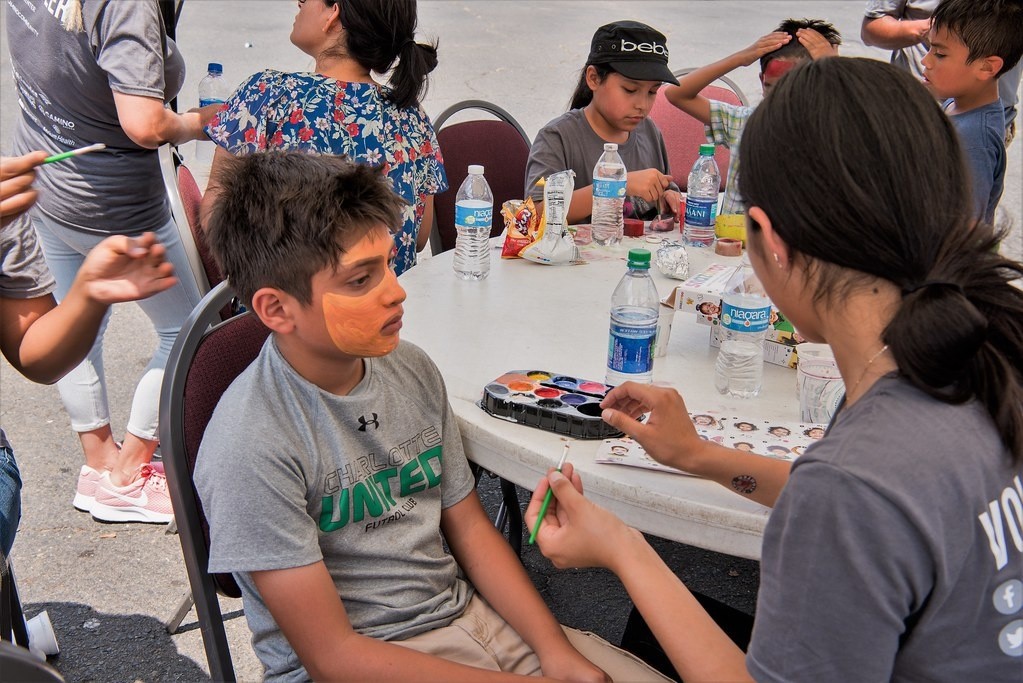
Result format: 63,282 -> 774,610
383,216 -> 846,564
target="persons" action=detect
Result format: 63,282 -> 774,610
524,53 -> 1022,683
7,0 -> 225,523
200,0 -> 450,315
734,422 -> 757,431
767,426 -> 791,438
861,0 -> 1023,144
692,415 -> 718,430
665,18 -> 841,214
609,446 -> 629,457
921,0 -> 1023,229
696,299 -> 722,320
767,445 -> 792,460
202,150 -> 675,683
521,20 -> 684,223
803,428 -> 826,439
768,309 -> 805,345
733,442 -> 754,452
0,150 -> 178,566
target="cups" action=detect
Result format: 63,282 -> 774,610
11,610 -> 59,656
797,361 -> 842,426
797,342 -> 833,405
655,304 -> 675,357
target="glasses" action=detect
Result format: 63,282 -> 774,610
297,0 -> 308,9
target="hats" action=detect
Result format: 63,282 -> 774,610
588,21 -> 680,87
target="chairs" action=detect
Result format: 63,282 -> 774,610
429,99 -> 531,256
159,143 -> 242,328
647,68 -> 749,192
156,278 -> 275,683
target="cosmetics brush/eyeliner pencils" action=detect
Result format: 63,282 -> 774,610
528,438 -> 572,543
33,143 -> 106,166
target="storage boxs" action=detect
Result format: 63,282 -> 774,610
664,261 -> 809,369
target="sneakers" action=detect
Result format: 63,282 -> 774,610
73,462 -> 166,512
91,461 -> 175,522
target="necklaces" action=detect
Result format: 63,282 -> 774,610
842,346 -> 888,407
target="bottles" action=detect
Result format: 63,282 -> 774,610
683,144 -> 720,246
712,253 -> 770,395
606,249 -> 659,389
453,165 -> 494,281
591,144 -> 627,245
196,63 -> 226,163
